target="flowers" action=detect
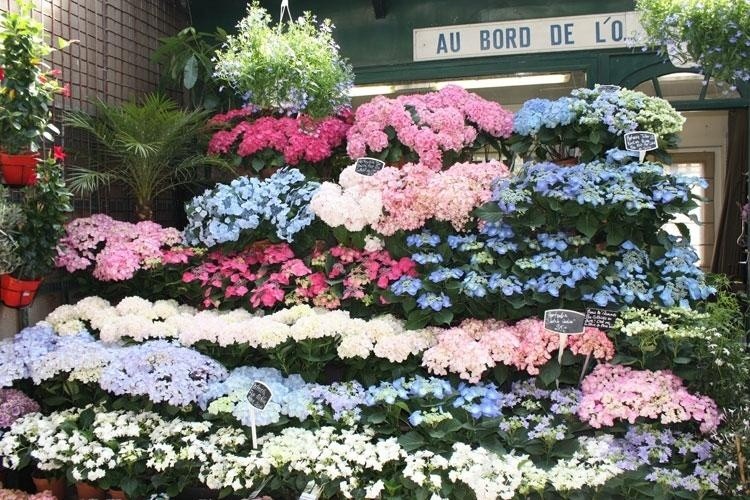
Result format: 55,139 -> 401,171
0,1 -> 749,500
